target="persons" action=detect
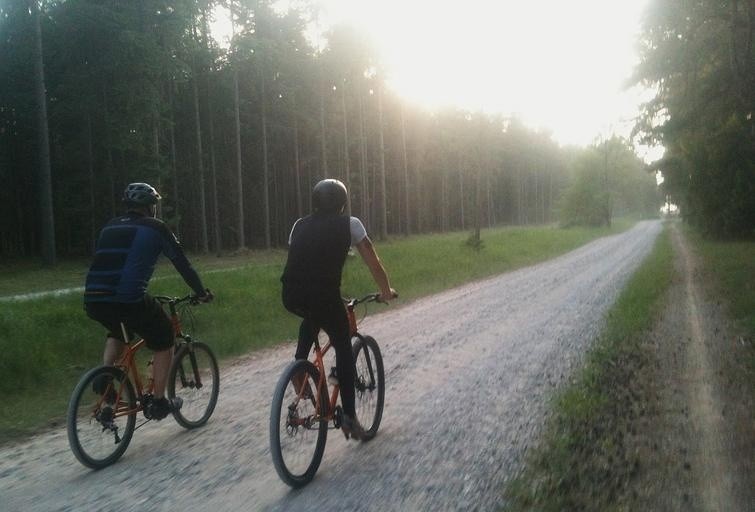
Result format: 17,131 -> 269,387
83,181 -> 207,420
281,177 -> 397,441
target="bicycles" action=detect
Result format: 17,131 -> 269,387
269,289 -> 399,489
67,289 -> 219,470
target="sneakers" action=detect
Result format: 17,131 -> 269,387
342,414 -> 365,441
93,374 -> 123,404
291,372 -> 313,400
143,396 -> 184,421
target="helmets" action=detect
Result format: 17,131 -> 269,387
121,182 -> 163,207
312,178 -> 347,212
